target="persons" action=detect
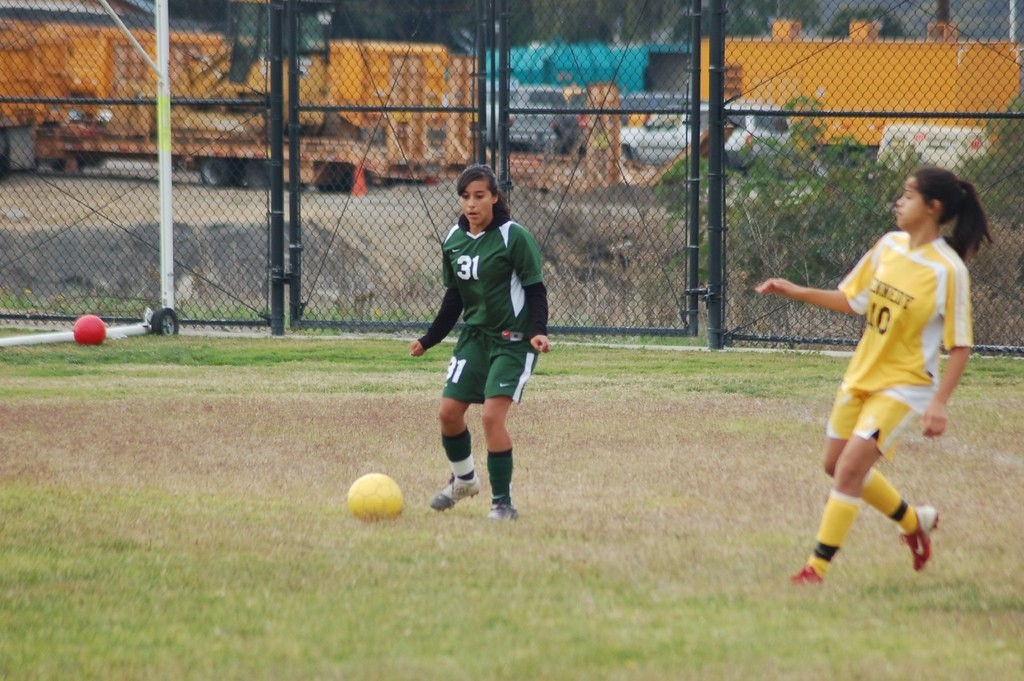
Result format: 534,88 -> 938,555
755,169 -> 994,585
410,163 -> 550,520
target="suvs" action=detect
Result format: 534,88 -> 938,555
618,94 -> 793,170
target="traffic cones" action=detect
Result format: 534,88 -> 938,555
351,160 -> 368,198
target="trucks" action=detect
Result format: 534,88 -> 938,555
0,18 -> 480,193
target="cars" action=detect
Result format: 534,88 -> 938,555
485,86 -> 567,155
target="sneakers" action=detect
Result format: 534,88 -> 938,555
486,504 -> 519,523
788,567 -> 823,586
899,507 -> 939,570
430,472 -> 481,510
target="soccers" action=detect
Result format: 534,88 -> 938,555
346,473 -> 405,526
73,314 -> 106,346
